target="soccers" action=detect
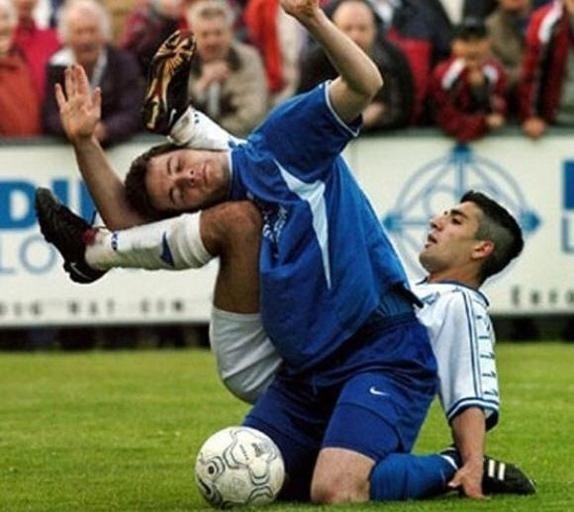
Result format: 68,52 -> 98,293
196,426 -> 285,509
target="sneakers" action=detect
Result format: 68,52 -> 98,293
32,185 -> 111,287
138,26 -> 200,138
440,441 -> 537,497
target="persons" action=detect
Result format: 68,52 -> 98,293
0,0 -> 573,343
